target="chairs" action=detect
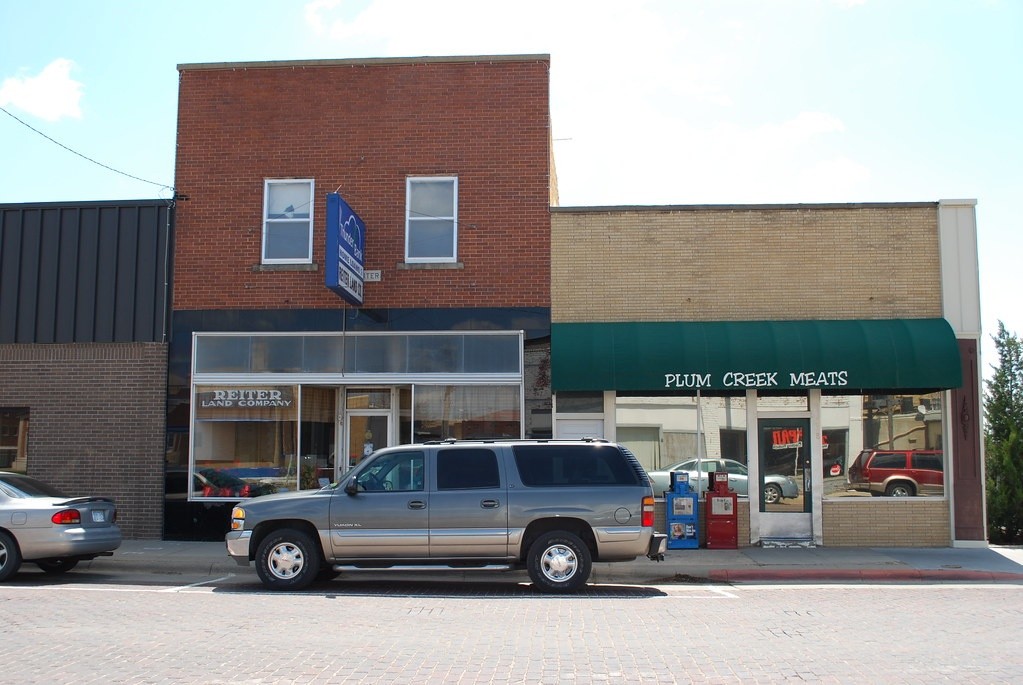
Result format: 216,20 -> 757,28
412,465 -> 424,488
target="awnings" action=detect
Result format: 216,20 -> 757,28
550,318 -> 963,394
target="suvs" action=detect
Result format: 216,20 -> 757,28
224,435 -> 667,592
850,448 -> 944,498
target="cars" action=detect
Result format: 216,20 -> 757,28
168,466 -> 271,497
647,457 -> 798,503
0,471 -> 119,582
